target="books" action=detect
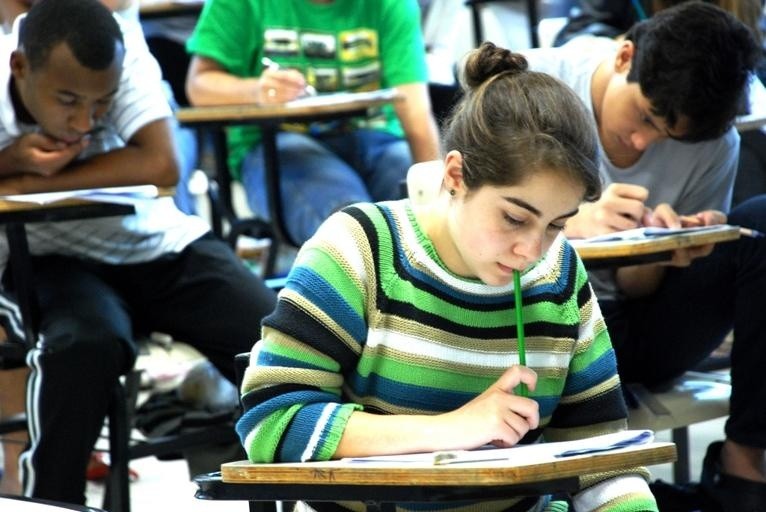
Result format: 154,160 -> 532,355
343,418 -> 653,468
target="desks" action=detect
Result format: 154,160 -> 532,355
563,224 -> 739,511
2,183 -> 185,511
195,439 -> 679,510
175,91 -> 413,280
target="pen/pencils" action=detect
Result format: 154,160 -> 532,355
680,215 -> 765,238
512,270 -> 529,398
261,56 -> 317,95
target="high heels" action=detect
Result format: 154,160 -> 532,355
700,442 -> 764,505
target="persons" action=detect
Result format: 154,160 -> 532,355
523,2 -> 766,483
232,39 -> 663,512
0,0 -> 765,377
1,2 -> 280,511
